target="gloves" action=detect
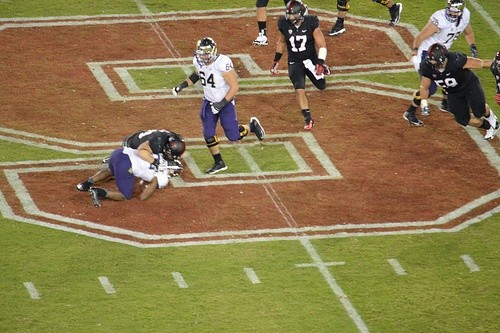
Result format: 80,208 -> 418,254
270,52 -> 283,74
149,159 -> 158,172
314,59 -> 324,75
420,99 -> 429,115
470,44 -> 477,58
172,81 -> 189,96
209,97 -> 227,114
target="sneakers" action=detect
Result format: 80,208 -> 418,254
439,104 -> 454,115
76,180 -> 89,192
403,111 -> 424,126
328,26 -> 345,35
304,119 -> 315,130
250,117 -> 265,141
483,108 -> 500,140
390,2 -> 402,26
323,64 -> 331,75
206,160 -> 228,174
89,187 -> 102,208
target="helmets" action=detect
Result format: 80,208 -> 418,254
195,37 -> 217,63
285,0 -> 306,18
425,43 -> 446,68
167,140 -> 186,157
444,0 -> 465,23
167,160 -> 183,178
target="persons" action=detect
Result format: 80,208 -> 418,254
418,43 -> 500,140
270,0 -> 332,131
252,0 -> 290,46
101,128 -> 185,171
173,38 -> 265,174
327,0 -> 402,35
491,51 -> 500,105
76,147 -> 183,207
404,0 -> 477,126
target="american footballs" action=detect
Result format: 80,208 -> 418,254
138,179 -> 152,193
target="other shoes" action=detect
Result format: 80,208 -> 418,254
252,32 -> 269,45
417,118 -> 419,119
